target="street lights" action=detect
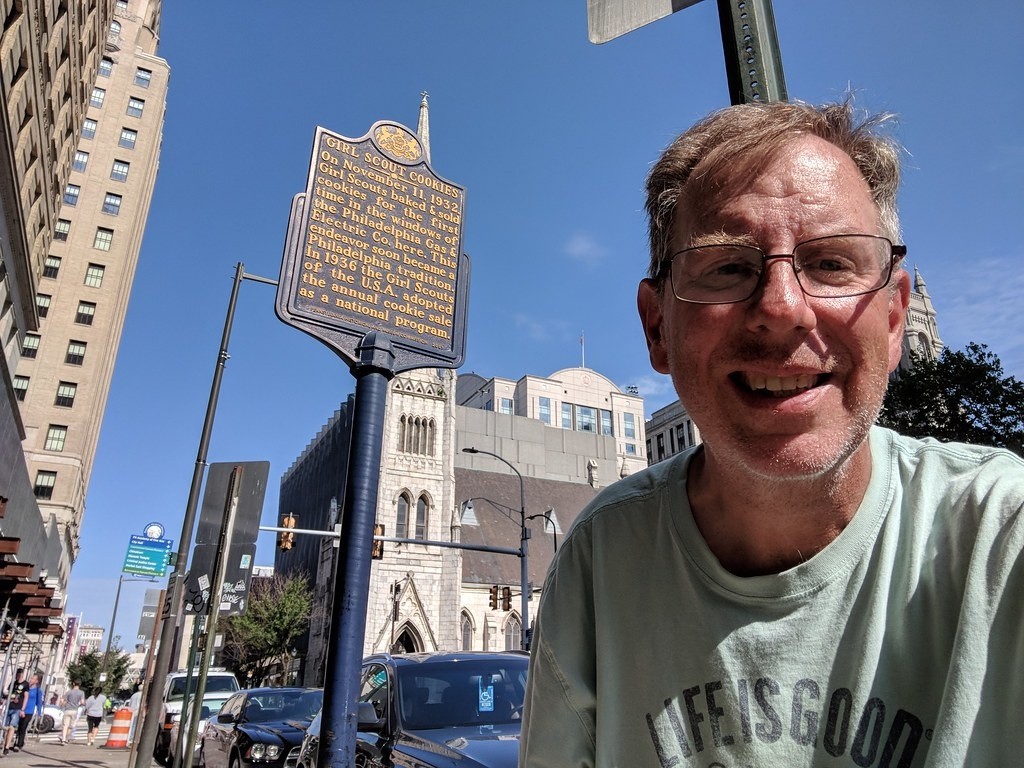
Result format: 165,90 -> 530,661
462,448 -> 528,650
525,513 -> 557,555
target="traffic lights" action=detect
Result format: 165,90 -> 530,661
502,587 -> 513,612
489,586 -> 498,611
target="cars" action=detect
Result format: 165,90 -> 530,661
167,690 -> 239,768
197,687 -> 326,768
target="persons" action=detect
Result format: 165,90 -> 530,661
12,673 -> 43,752
85,686 -> 106,746
518,84 -> 1024,768
59,678 -> 85,745
2,668 -> 29,756
130,685 -> 143,743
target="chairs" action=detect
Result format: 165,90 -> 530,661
405,684 -> 521,729
244,701 -> 314,723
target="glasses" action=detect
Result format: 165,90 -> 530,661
658,233 -> 909,305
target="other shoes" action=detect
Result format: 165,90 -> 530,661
14,747 -> 20,752
3,748 -> 9,756
60,741 -> 68,746
91,740 -> 94,745
87,743 -> 91,746
9,747 -> 14,750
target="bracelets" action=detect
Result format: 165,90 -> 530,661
21,708 -> 24,711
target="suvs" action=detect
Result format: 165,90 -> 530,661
151,667 -> 240,760
1,696 -> 65,733
294,650 -> 533,768
